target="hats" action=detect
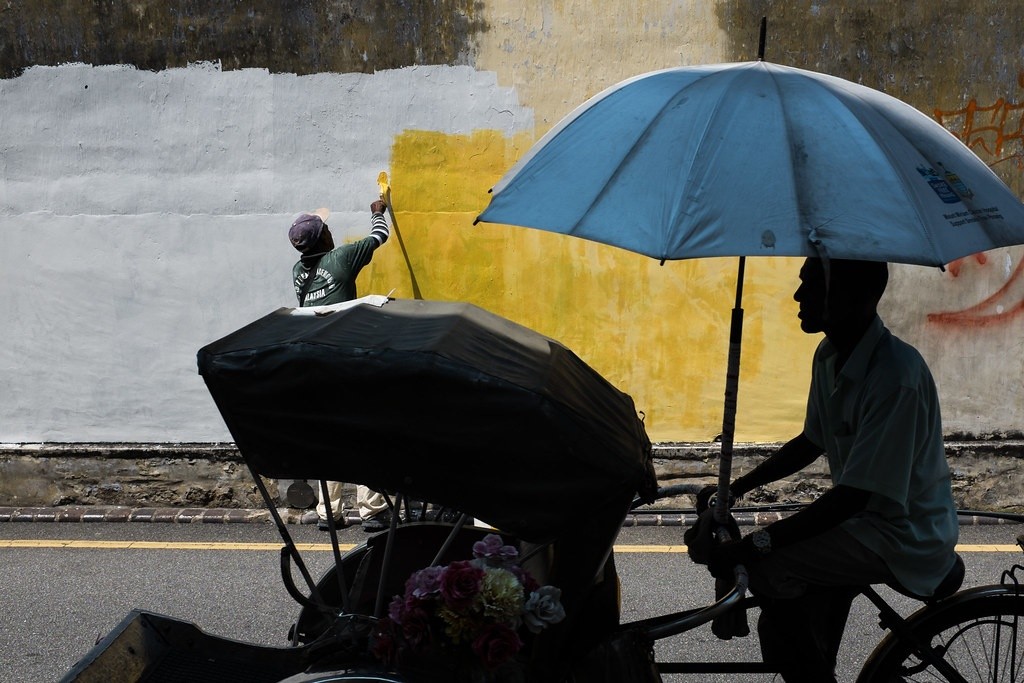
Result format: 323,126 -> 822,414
288,207 -> 332,250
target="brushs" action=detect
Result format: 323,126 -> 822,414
379,182 -> 389,201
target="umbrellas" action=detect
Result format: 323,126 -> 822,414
473,12 -> 1024,611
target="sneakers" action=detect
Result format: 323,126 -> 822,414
316,516 -> 346,528
361,509 -> 393,528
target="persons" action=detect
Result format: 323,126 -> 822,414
289,191 -> 393,530
699,254 -> 960,682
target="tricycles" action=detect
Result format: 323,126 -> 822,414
50,14 -> 1024,683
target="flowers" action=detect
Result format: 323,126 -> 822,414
367,530 -> 568,676
